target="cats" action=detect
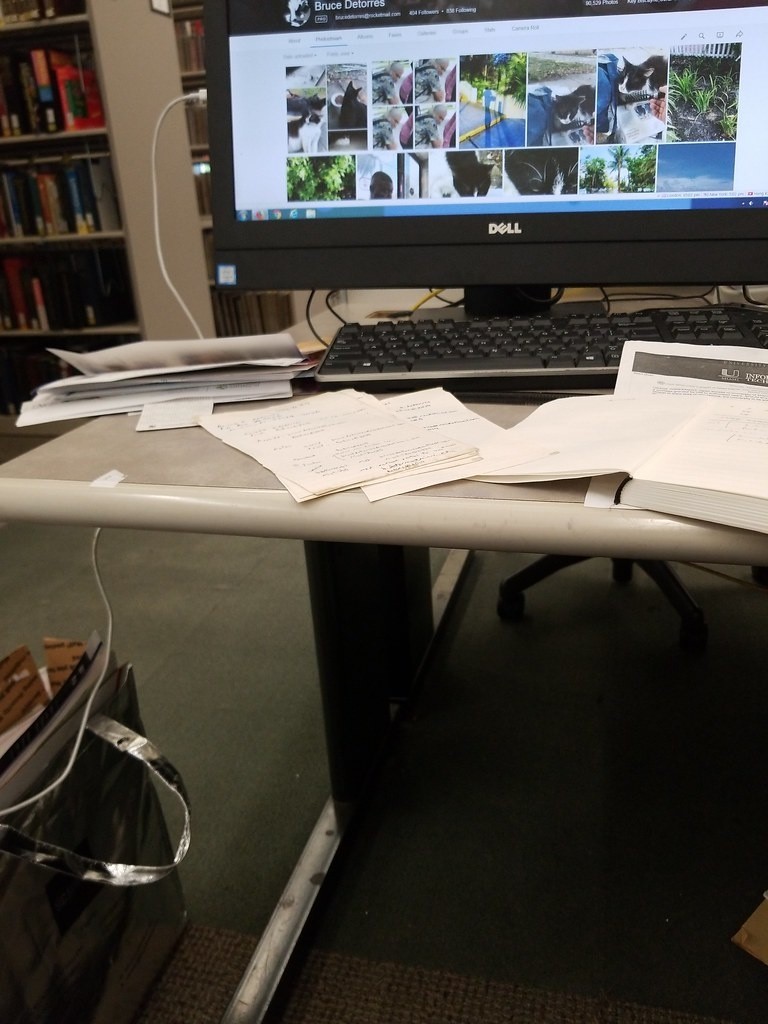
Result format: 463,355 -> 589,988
555,84 -> 595,124
340,81 -> 367,128
618,55 -> 669,95
288,110 -> 324,155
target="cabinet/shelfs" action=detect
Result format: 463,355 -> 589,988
0,0 -> 339,435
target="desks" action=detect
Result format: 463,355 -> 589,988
0,296 -> 768,1024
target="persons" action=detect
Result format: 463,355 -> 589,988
415,58 -> 449,103
416,104 -> 447,149
372,108 -> 402,151
372,62 -> 406,105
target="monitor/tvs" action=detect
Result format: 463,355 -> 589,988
201,0 -> 768,321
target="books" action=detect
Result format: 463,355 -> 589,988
36,333 -> 309,411
468,394 -> 768,534
0,0 -> 294,417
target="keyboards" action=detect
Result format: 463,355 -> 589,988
314,310 -> 768,393
